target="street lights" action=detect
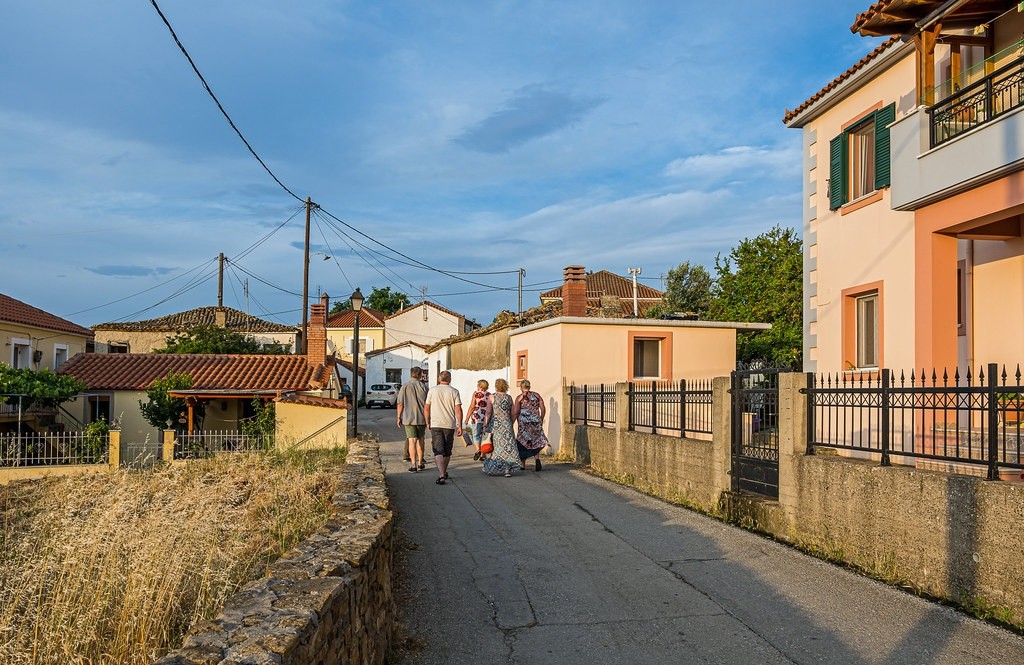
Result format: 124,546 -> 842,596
350,284 -> 365,438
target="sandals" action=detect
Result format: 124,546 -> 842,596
436,471 -> 448,485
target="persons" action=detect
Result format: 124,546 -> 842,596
423,371 -> 463,484
464,380 -> 493,461
403,436 -> 427,464
397,366 -> 431,473
483,378 -> 514,477
512,379 -> 546,472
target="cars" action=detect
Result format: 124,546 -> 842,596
338,383 -> 353,404
366,383 -> 403,408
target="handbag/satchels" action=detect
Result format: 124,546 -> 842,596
461,424 -> 472,447
481,432 -> 493,453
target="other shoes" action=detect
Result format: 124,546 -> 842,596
403,458 -> 411,462
473,449 -> 487,461
408,467 -> 417,473
520,465 -> 526,470
535,459 -> 542,471
422,458 -> 426,463
504,473 -> 511,477
418,463 -> 425,470
